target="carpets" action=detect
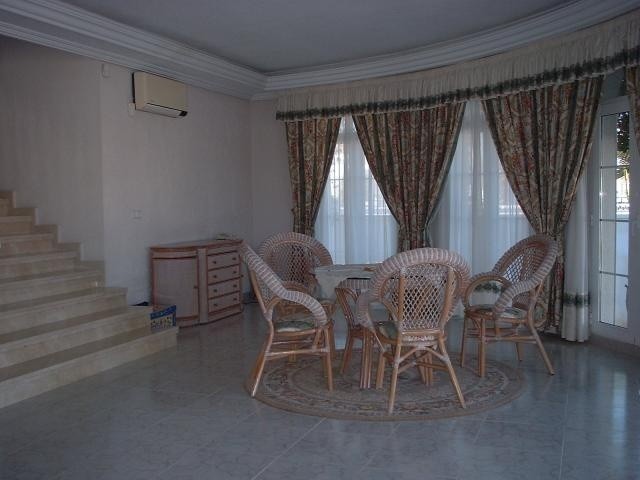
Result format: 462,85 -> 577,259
249,346 -> 526,422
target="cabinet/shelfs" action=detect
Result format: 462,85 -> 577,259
149,237 -> 245,329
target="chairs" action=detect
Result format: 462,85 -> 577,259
237,243 -> 337,399
353,246 -> 467,418
460,234 -> 558,379
258,231 -> 339,380
335,287 -> 402,390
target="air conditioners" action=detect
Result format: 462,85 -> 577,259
131,71 -> 188,117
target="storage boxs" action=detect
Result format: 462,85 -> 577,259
134,301 -> 177,329
